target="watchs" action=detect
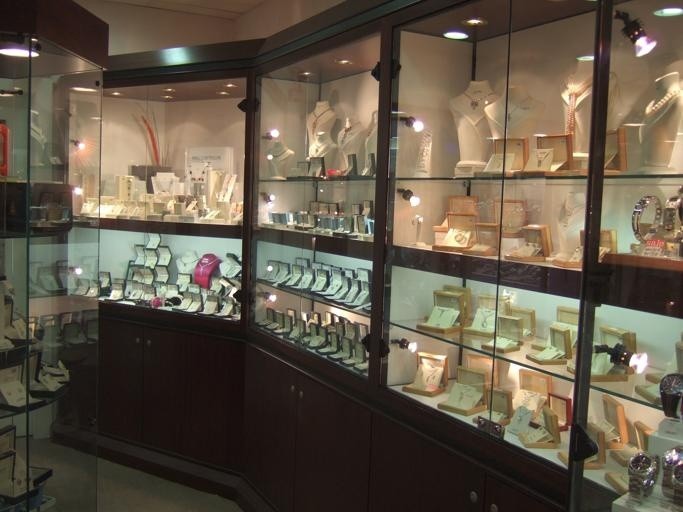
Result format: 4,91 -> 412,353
662,445 -> 683,505
660,372 -> 683,419
628,452 -> 661,503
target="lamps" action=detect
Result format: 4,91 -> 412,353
399,116 -> 424,132
262,193 -> 276,203
397,188 -> 421,207
263,292 -> 277,303
265,129 -> 279,141
391,338 -> 417,354
595,343 -> 650,375
614,9 -> 657,58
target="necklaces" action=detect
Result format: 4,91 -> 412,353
331,280 -> 340,291
367,123 -> 378,137
462,92 -> 496,110
223,175 -> 232,201
337,287 -> 348,295
502,205 -> 521,228
536,150 -> 552,168
154,176 -> 173,193
188,166 -> 208,181
500,95 -> 531,122
479,309 -> 495,328
356,286 -> 368,303
560,198 -> 586,227
420,365 -> 439,382
456,382 -> 476,405
312,108 -> 333,135
198,258 -> 219,276
303,273 -> 312,285
514,406 -> 533,426
180,256 -> 200,267
290,272 -> 300,283
345,285 -> 357,301
31,127 -> 46,142
438,305 -> 453,318
315,278 -> 325,288
276,268 -> 286,282
341,121 -> 361,144
643,89 -> 681,118
523,388 -> 538,404
221,262 -> 238,277
132,289 -> 141,296
271,148 -> 288,157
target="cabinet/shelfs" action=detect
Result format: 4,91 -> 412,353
368,409 -> 568,512
52,311 -> 244,496
246,343 -> 372,512
50,39 -> 250,326
248,1 -> 683,474
0,1 -> 109,512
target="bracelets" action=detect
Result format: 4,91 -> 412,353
664,197 -> 683,242
632,195 -> 662,244
